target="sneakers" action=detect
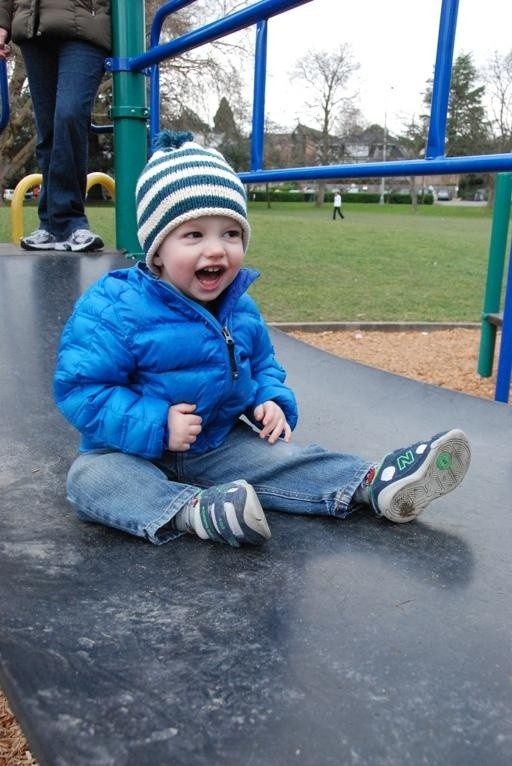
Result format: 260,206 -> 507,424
54,229 -> 104,253
19,229 -> 56,251
186,478 -> 271,550
364,427 -> 471,526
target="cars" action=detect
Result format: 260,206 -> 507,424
303,186 -> 452,203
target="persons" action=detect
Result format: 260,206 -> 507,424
0,0 -> 138,255
332,190 -> 345,220
52,130 -> 472,550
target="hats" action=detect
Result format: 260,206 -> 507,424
134,126 -> 250,277
